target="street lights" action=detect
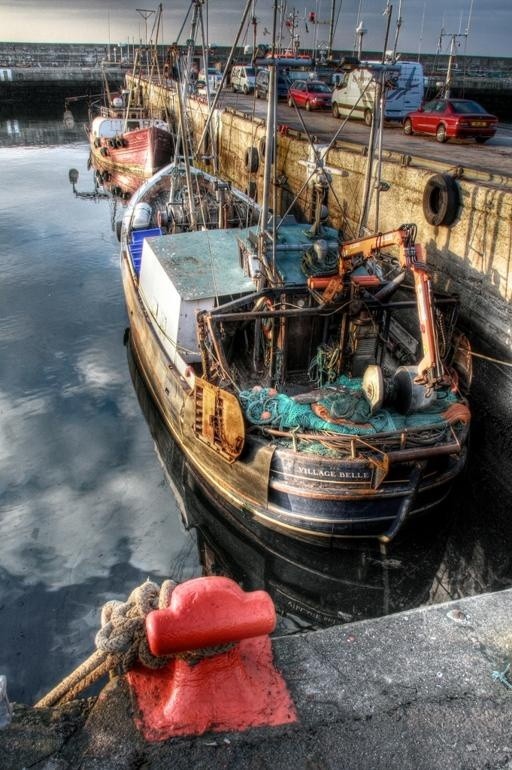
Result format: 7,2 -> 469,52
353,17 -> 367,60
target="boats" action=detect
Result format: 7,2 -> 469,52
110,0 -> 505,560
88,1 -> 197,197
114,317 -> 468,629
69,151 -> 130,210
